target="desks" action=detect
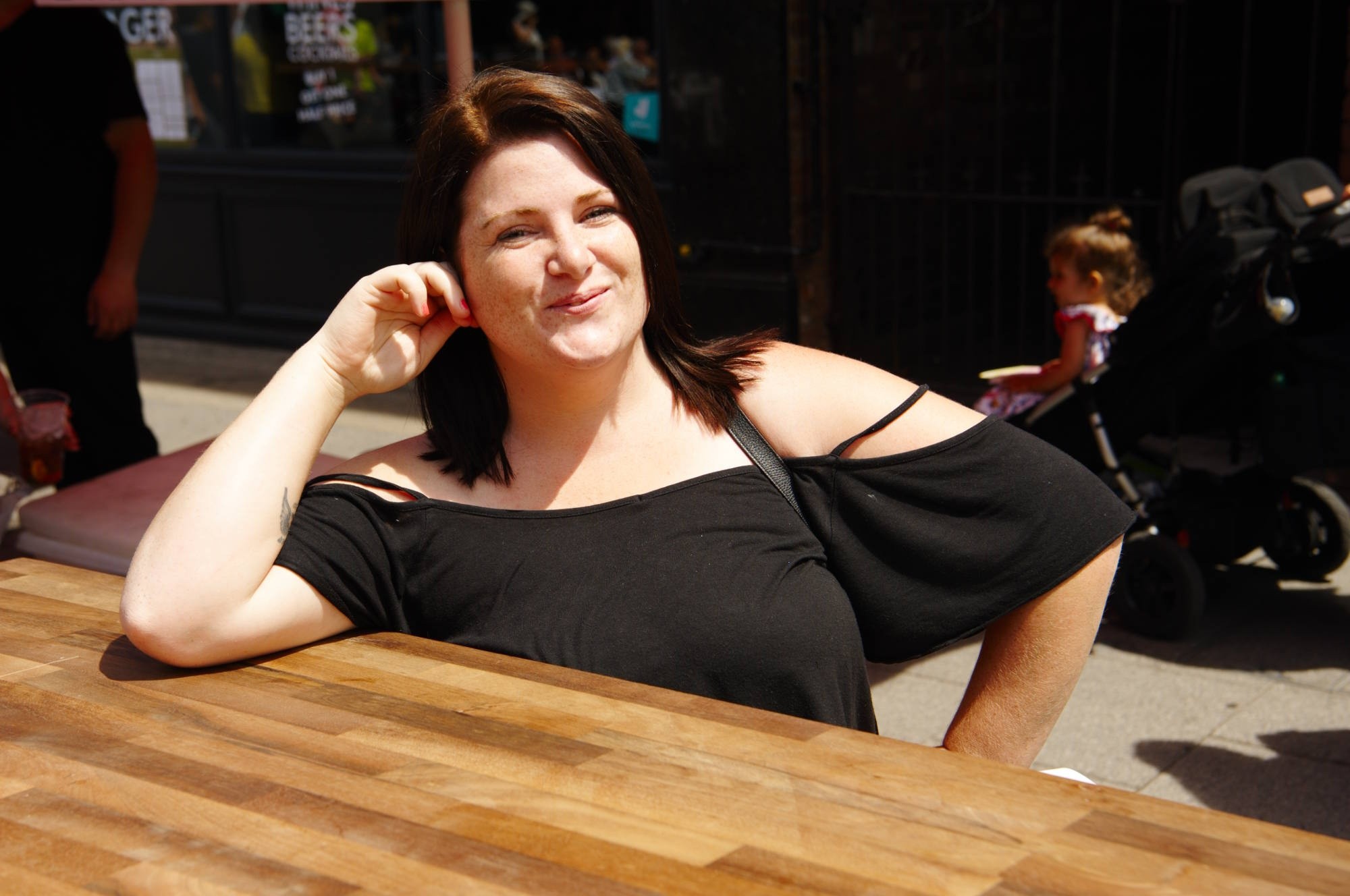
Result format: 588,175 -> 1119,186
1,552 -> 1350,895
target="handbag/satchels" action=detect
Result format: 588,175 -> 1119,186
621,81 -> 663,141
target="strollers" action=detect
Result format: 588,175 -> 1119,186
912,163 -> 1350,643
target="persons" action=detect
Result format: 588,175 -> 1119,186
0,0 -> 159,490
973,204 -> 1151,418
119,65 -> 1136,766
500,0 -> 658,91
175,6 -> 226,148
